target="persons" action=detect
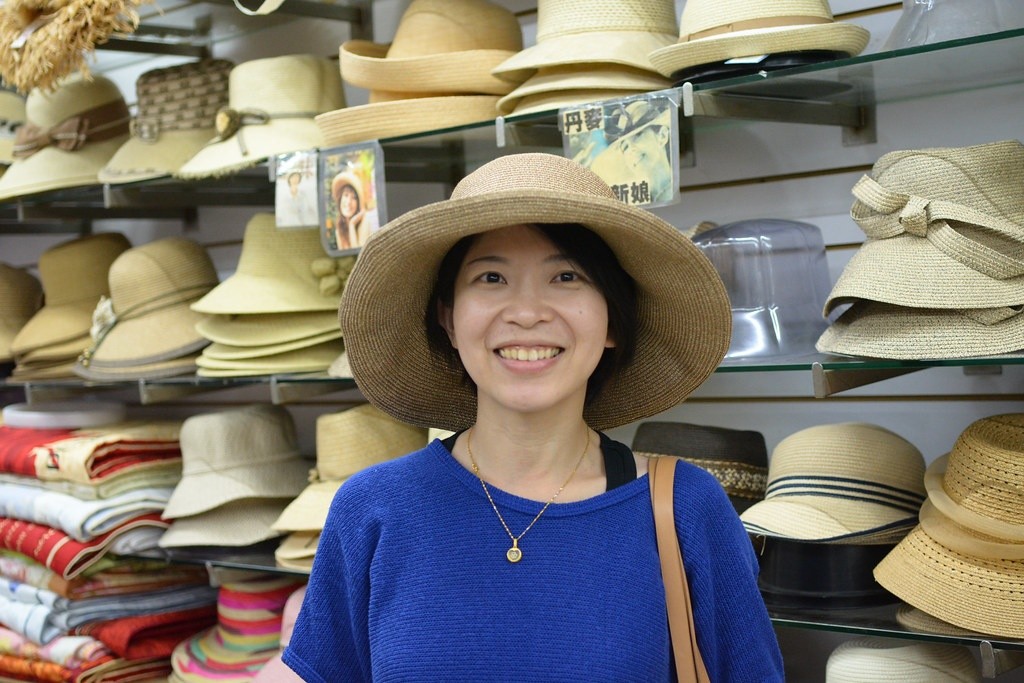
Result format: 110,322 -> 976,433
573,101 -> 673,203
280,153 -> 785,683
280,167 -> 316,224
332,172 -> 372,250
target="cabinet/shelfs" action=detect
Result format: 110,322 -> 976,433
0,0 -> 1024,683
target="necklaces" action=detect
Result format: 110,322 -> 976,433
468,421 -> 590,562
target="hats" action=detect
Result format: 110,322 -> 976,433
332,166 -> 366,211
0,91 -> 28,166
0,208 -> 361,385
286,168 -> 304,183
172,51 -> 350,181
95,57 -> 236,185
0,71 -> 133,200
628,415 -> 1024,683
589,100 -> 671,188
312,0 -> 871,149
814,140 -> 1024,362
337,153 -> 734,433
160,389 -> 429,683
